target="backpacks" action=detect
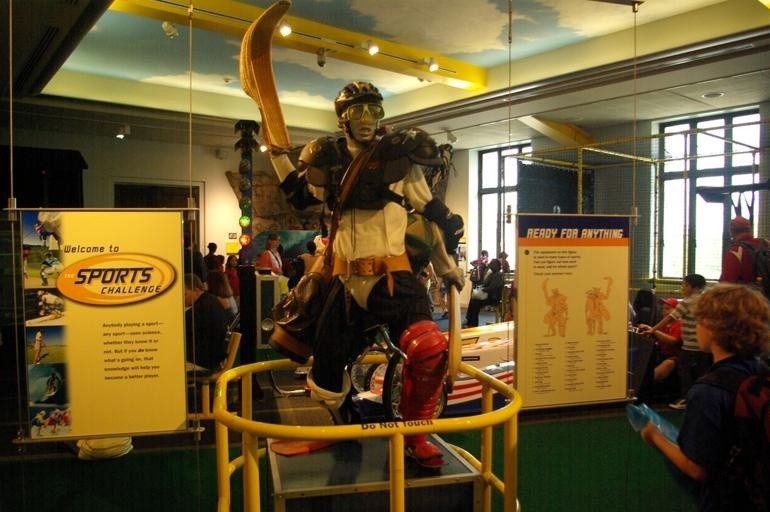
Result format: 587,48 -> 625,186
733,236 -> 770,293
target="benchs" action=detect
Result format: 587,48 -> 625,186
189,334 -> 242,423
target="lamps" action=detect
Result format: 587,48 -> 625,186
83,2 -> 445,83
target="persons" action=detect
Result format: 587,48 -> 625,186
637,296 -> 684,393
426,250 -> 511,323
37,288 -> 63,317
29,372 -> 70,434
185,237 -> 320,371
34,223 -> 49,241
638,275 -> 710,408
24,245 -> 30,278
33,331 -> 43,365
641,283 -> 770,511
39,251 -> 64,284
718,214 -> 770,284
270,80 -> 467,487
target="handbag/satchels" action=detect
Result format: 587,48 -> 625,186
471,286 -> 489,300
470,267 -> 483,282
